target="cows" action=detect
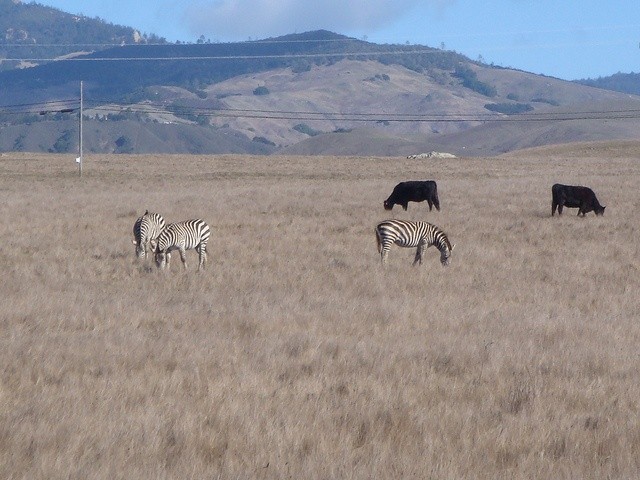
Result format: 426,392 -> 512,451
549,183 -> 607,218
382,179 -> 441,213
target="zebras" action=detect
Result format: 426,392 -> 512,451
151,218 -> 215,270
129,210 -> 166,261
372,217 -> 458,269
